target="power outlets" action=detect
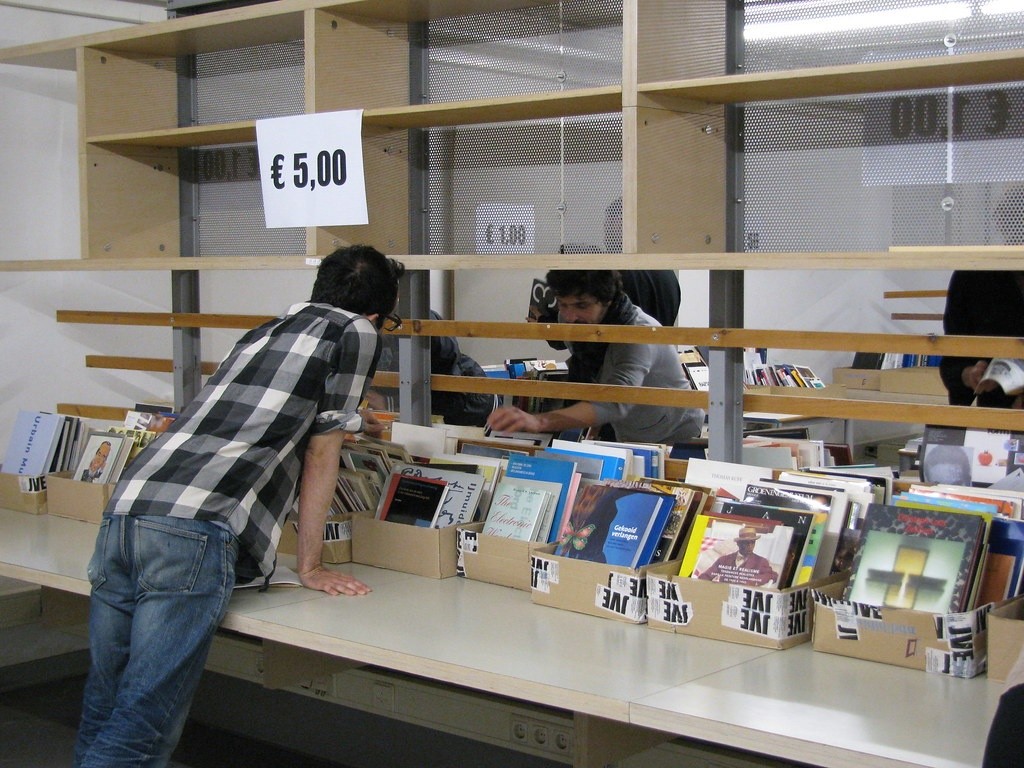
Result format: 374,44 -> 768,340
508,713 -> 573,757
254,650 -> 338,698
370,679 -> 394,711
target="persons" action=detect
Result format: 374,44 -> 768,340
76,244 -> 402,768
363,303 -> 500,427
698,527 -> 778,588
487,243 -> 704,444
940,185 -> 1024,409
524,198 -> 680,441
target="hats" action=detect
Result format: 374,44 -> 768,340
735,527 -> 760,543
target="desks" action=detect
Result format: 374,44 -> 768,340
747,411 -> 854,465
0,508 -> 1024,768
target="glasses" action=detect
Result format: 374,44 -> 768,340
378,313 -> 402,332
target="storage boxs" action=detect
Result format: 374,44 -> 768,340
985,599 -> 1024,682
831,367 -> 880,390
646,559 -> 844,647
452,523 -> 554,591
0,471 -> 45,513
530,542 -> 676,626
276,513 -> 352,564
46,472 -> 107,525
881,368 -> 949,397
812,580 -> 994,680
349,511 -> 471,579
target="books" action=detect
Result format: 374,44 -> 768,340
2,346 -> 1024,611
